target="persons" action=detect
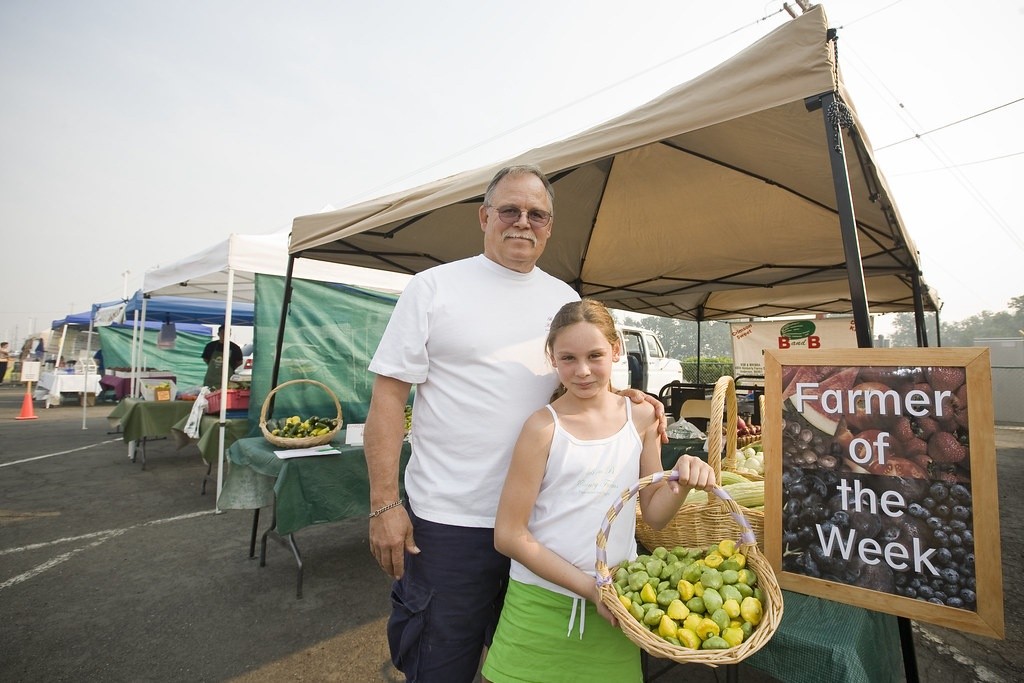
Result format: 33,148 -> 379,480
362,164 -> 668,683
0,342 -> 9,387
200,324 -> 243,392
481,299 -> 715,683
93,349 -> 105,379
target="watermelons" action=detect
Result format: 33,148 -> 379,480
782,366 -> 860,435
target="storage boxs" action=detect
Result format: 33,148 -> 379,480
140,379 -> 178,401
205,388 -> 251,413
105,369 -> 172,378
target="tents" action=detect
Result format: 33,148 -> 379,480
249,2 -> 943,683
19,227 -> 417,515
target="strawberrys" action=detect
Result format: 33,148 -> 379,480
891,366 -> 970,483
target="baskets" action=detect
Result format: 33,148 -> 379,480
704,426 -> 762,451
595,470 -> 784,668
634,376 -> 764,558
257,379 -> 344,446
734,395 -> 766,480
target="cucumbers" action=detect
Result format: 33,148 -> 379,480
272,416 -> 337,438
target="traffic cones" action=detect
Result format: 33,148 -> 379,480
15,379 -> 39,420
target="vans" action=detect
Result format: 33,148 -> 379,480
611,322 -> 683,406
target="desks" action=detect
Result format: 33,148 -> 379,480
32,371 -> 102,408
99,375 -> 176,402
659,438 -> 726,469
640,589 -> 906,683
106,397 -> 195,470
168,413 -> 248,494
217,430 -> 411,599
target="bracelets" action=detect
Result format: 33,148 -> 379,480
367,499 -> 403,518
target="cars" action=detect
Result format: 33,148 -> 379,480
231,341 -> 320,384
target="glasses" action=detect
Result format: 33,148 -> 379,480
486,206 -> 555,228
